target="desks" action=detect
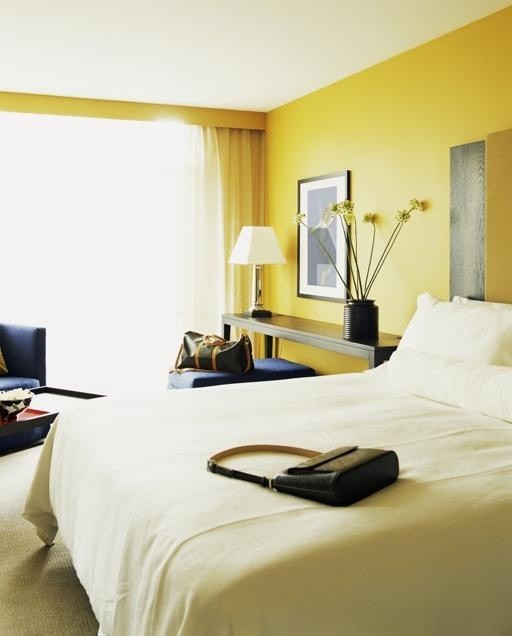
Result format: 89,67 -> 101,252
221,311 -> 404,369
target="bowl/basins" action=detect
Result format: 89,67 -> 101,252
0,390 -> 37,421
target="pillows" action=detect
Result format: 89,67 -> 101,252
390,295 -> 512,365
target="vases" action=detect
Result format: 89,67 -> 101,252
344,299 -> 378,343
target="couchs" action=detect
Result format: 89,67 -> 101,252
0,323 -> 51,457
166,359 -> 314,389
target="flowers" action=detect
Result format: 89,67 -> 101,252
294,198 -> 424,300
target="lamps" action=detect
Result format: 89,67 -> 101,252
228,226 -> 288,318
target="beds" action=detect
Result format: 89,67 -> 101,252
22,296 -> 511,636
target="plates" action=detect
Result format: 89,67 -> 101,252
0,386 -> 110,428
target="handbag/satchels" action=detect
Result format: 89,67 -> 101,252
173,330 -> 255,377
206,444 -> 400,508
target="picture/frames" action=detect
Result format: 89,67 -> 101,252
297,170 -> 349,304
